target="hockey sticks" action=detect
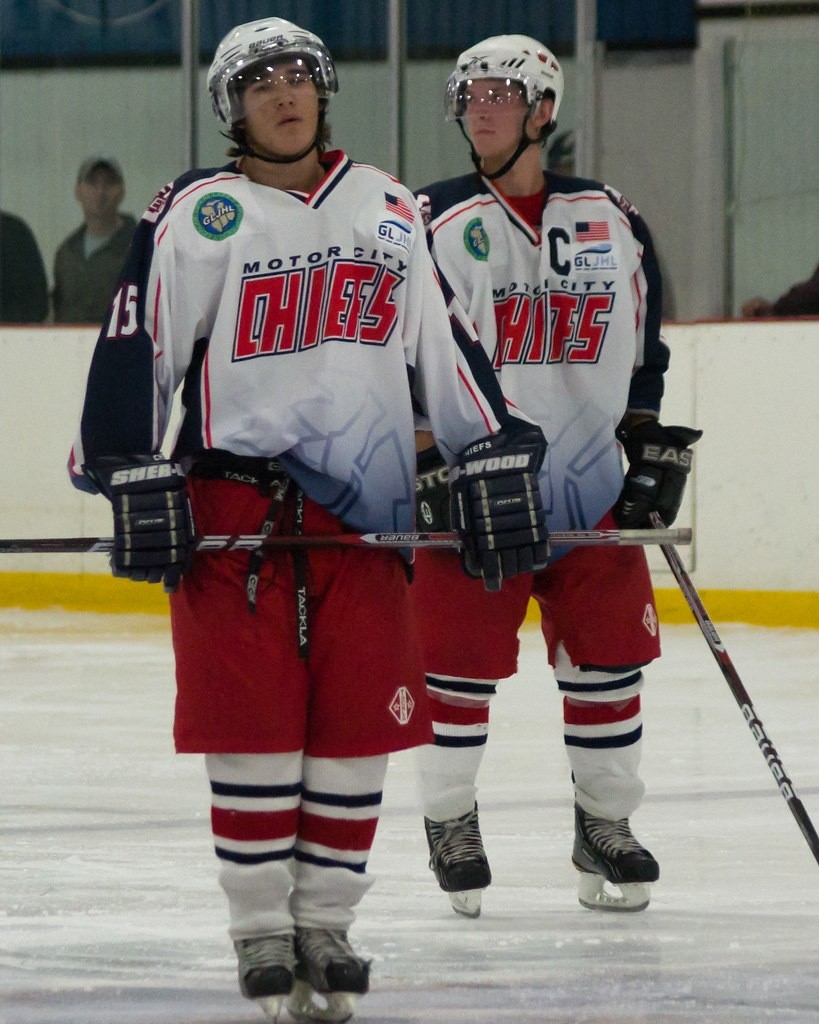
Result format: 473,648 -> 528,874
651,508 -> 819,863
2,529 -> 693,553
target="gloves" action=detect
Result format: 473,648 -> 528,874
79,451 -> 196,595
452,423 -> 550,594
608,423 -> 706,527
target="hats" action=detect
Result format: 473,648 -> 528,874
76,153 -> 125,189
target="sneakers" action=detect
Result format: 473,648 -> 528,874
423,799 -> 492,919
232,927 -> 376,1023
572,793 -> 661,915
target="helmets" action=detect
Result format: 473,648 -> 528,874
442,33 -> 563,138
206,16 -> 339,131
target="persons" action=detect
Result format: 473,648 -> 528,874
0,211 -> 48,324
68,18 -> 553,999
44,154 -> 147,326
413,36 -> 705,892
741,263 -> 817,317
